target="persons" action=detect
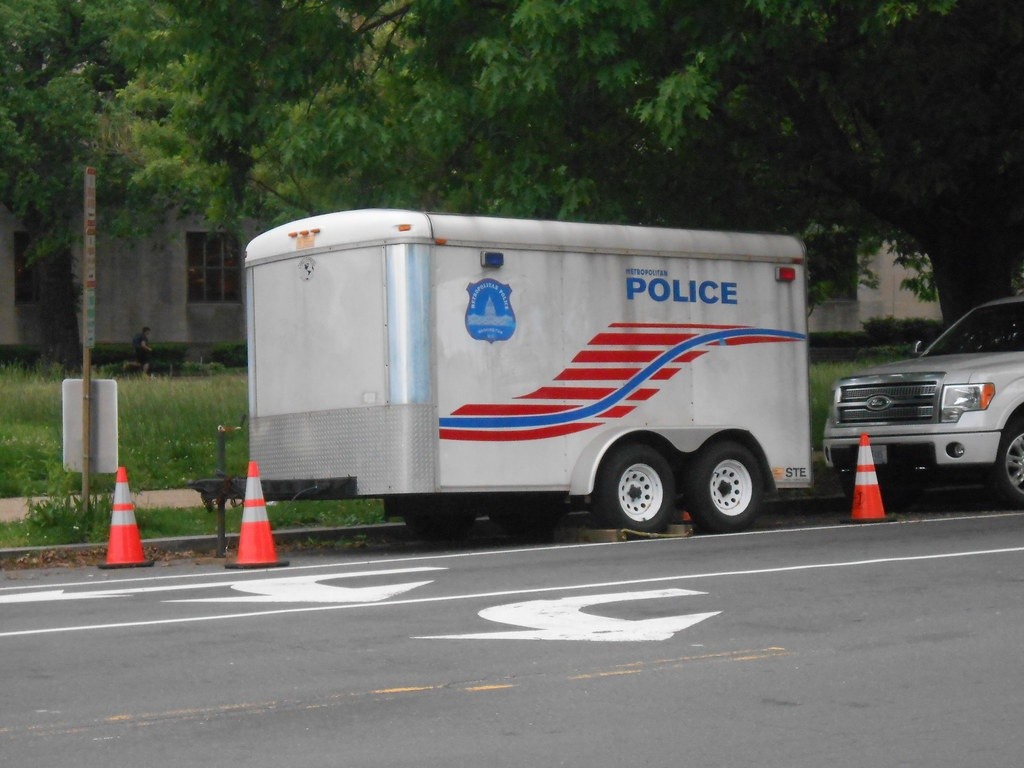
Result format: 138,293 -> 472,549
134,327 -> 152,379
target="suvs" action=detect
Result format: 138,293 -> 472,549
823,295 -> 1024,511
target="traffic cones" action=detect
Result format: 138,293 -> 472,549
95,466 -> 158,568
225,461 -> 291,569
839,432 -> 898,523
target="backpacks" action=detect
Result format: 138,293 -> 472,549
133,335 -> 142,352
